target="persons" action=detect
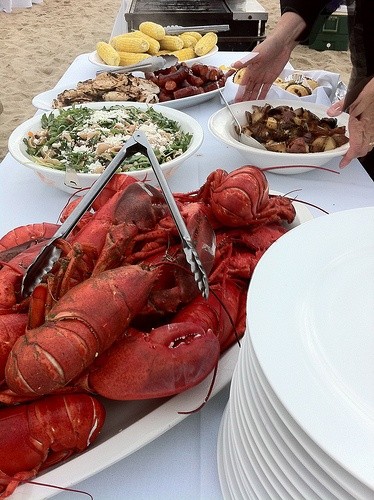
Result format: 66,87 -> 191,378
225,0 -> 374,170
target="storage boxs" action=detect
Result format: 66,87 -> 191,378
309,5 -> 349,51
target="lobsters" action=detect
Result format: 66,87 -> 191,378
0,164 -> 340,500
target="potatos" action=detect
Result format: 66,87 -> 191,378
232,104 -> 350,153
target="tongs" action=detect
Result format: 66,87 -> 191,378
164,24 -> 230,36
93,54 -> 178,76
16,132 -> 210,305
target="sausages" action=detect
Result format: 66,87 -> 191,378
145,62 -> 236,102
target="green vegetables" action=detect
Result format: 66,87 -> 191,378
23,103 -> 194,170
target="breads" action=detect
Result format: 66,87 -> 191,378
234,67 -> 319,96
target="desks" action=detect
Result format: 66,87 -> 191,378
0,52 -> 374,500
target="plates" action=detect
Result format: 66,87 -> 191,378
213,205 -> 374,499
33,66 -> 229,111
88,43 -> 219,72
1,189 -> 310,499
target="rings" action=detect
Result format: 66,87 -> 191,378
369,142 -> 373,145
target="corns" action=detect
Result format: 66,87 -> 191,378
97,22 -> 217,66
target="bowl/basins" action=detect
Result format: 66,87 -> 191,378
7,101 -> 205,196
208,100 -> 358,175
